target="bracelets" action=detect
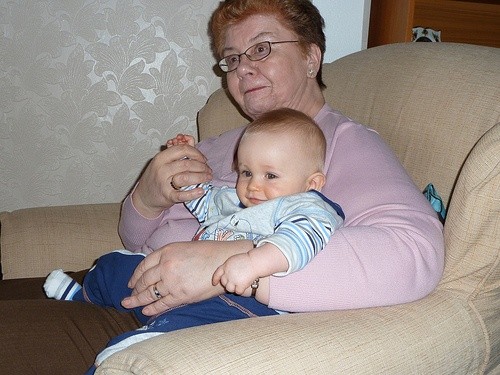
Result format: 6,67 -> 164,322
250,277 -> 259,299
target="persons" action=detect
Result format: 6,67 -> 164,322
43,106 -> 346,375
0,0 -> 445,375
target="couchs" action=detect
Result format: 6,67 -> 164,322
0,42 -> 500,375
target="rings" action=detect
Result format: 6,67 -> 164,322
170,174 -> 182,190
153,282 -> 162,300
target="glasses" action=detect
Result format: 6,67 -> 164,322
218,40 -> 300,73
